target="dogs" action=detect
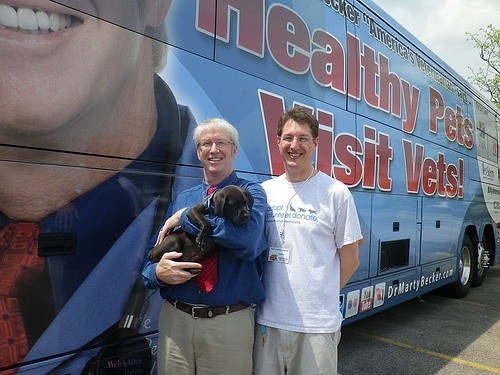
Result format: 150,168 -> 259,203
148,186 -> 254,275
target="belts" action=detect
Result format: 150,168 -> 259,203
166,296 -> 251,319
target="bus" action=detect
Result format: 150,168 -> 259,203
0,1 -> 500,375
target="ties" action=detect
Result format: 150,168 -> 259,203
196,187 -> 219,294
0,222 -> 46,375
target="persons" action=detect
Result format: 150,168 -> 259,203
0,0 -> 203,375
252,104 -> 363,375
141,117 -> 267,375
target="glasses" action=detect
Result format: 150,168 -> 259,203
197,139 -> 235,150
